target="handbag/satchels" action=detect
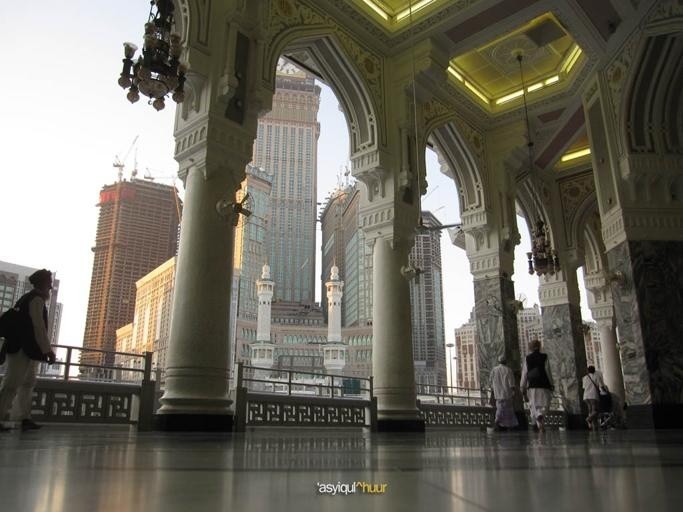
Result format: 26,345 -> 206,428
0,309 -> 18,340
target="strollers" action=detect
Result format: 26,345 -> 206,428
599,386 -> 629,430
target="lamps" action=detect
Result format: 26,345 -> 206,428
511,49 -> 561,280
119,1 -> 189,111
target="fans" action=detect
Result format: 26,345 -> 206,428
391,1 -> 465,236
216,186 -> 256,228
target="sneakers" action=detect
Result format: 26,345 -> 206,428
0,420 -> 41,431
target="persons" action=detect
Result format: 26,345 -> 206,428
579,364 -> 610,436
488,353 -> 520,433
0,268 -> 59,436
518,340 -> 556,435
